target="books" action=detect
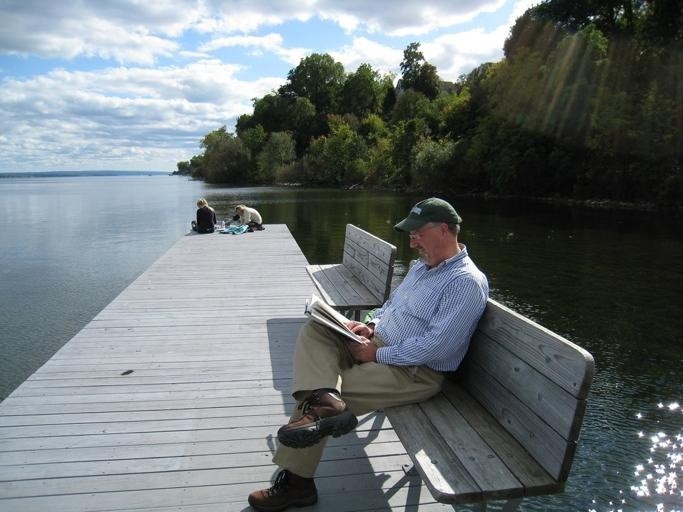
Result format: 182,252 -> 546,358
302,292 -> 367,346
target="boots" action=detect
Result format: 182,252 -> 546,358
248,468 -> 318,511
277,389 -> 358,448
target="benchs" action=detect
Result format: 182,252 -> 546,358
375,295 -> 595,512
306,223 -> 397,321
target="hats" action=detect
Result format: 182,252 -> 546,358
393,196 -> 465,237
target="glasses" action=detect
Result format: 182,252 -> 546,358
408,225 -> 439,241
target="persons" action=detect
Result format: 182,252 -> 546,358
248,198 -> 489,510
191,198 -> 217,233
225,204 -> 263,232
